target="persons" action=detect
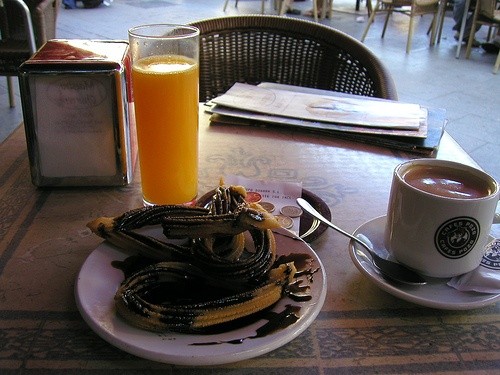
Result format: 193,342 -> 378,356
303,0 -> 331,17
452,0 -> 500,53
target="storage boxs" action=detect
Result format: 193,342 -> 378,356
18,37 -> 136,188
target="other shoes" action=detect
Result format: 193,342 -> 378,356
481,42 -> 500,55
453,30 -> 481,48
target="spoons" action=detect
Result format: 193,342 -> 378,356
296,196 -> 427,286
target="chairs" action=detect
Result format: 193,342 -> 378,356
34,0 -> 57,44
149,14 -> 397,102
0,0 -> 36,107
465,0 -> 500,74
361,0 -> 441,54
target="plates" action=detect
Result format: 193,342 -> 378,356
195,182 -> 331,244
74,223 -> 327,364
348,214 -> 500,310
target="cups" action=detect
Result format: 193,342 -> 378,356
128,23 -> 200,207
383,159 -> 499,279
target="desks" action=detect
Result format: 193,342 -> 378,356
0,105 -> 499,374
